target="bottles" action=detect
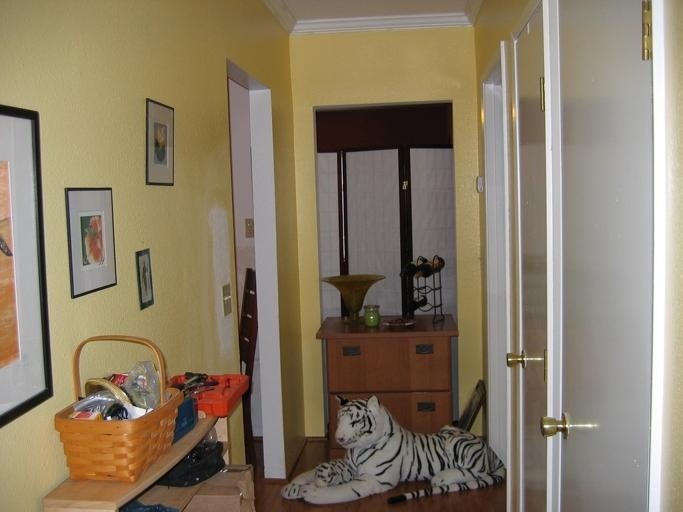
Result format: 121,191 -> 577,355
364,305 -> 380,328
399,257 -> 444,315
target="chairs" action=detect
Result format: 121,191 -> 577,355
239,268 -> 258,447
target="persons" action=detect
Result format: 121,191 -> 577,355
141,260 -> 150,295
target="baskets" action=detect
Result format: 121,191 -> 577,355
49,333 -> 185,484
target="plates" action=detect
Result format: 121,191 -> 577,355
384,320 -> 416,328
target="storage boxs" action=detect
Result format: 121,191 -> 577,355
181,464 -> 257,512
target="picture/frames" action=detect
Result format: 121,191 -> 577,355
459,379 -> 486,431
65,187 -> 117,299
0,104 -> 53,428
135,248 -> 154,310
145,98 -> 175,187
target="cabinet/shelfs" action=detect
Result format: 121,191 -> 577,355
42,415 -> 229,512
316,314 -> 459,458
